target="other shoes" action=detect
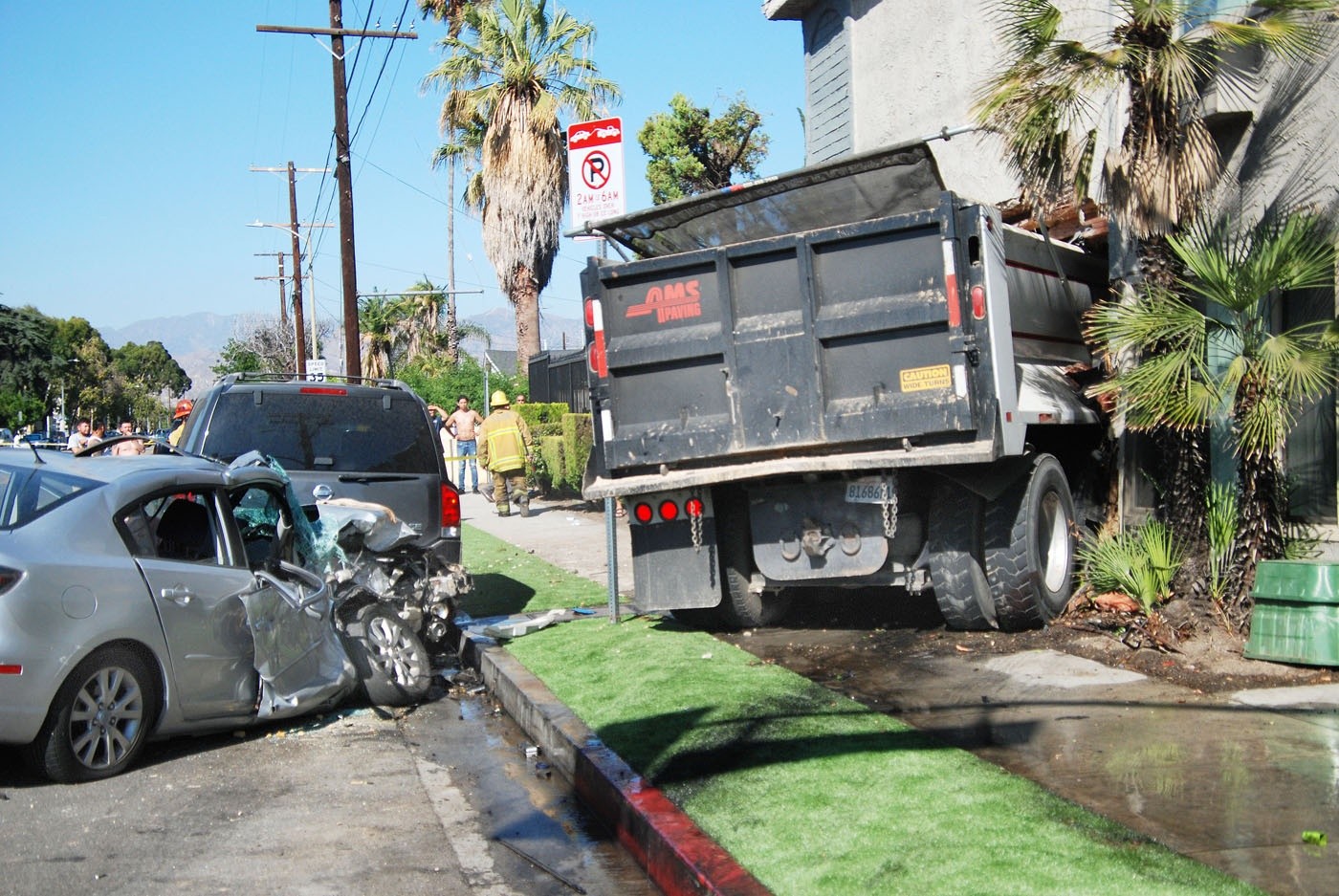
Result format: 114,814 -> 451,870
457,488 -> 465,495
473,489 -> 481,494
481,488 -> 495,503
508,492 -> 513,498
519,495 -> 529,517
615,508 -> 627,518
498,511 -> 511,517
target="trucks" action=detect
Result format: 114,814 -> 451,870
563,124 -> 1107,634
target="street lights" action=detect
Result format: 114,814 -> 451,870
245,221 -> 317,361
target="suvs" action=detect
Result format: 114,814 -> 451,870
174,372 -> 461,565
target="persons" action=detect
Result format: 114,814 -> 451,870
87,419 -> 105,458
67,417 -> 91,492
444,395 -> 483,495
112,422 -> 145,456
427,404 -> 449,438
477,390 -> 534,518
168,400 -> 195,500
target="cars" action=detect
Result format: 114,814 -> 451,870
0,435 -> 469,788
0,428 -> 67,450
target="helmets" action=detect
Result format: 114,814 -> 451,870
490,390 -> 509,406
173,400 -> 193,419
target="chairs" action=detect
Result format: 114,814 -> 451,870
155,498 -> 211,563
